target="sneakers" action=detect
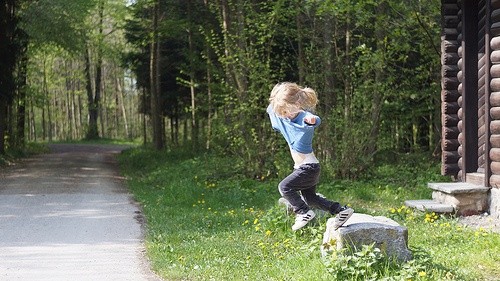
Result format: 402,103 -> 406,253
331,205 -> 355,230
290,210 -> 315,230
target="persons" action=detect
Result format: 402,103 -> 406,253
266,81 -> 355,231
